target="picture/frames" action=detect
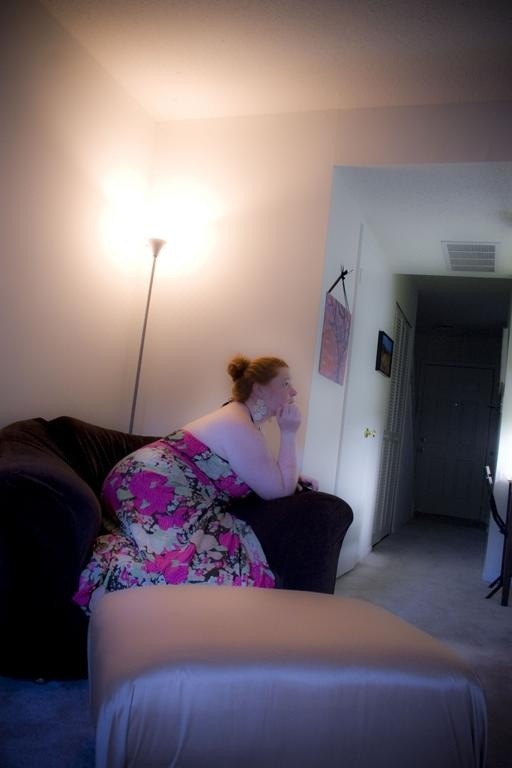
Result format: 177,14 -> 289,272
374,329 -> 394,378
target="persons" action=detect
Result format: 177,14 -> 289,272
71,352 -> 320,619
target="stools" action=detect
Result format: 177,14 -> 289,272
87,584 -> 488,768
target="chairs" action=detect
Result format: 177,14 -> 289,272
1,416 -> 354,684
482,463 -> 512,599
